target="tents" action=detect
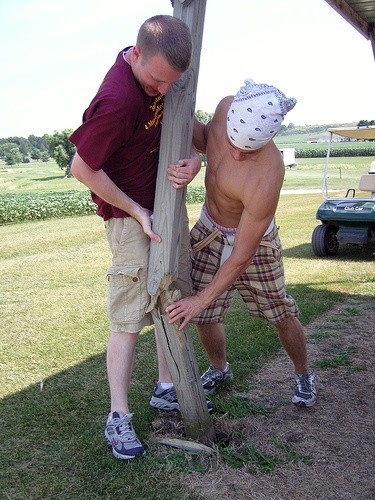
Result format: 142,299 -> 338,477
322,127 -> 375,197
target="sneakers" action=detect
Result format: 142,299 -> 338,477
104,410 -> 146,460
292,372 -> 316,408
200,362 -> 233,394
150,380 -> 213,412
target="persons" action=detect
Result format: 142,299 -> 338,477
67,16 -> 213,459
165,78 -> 317,408
